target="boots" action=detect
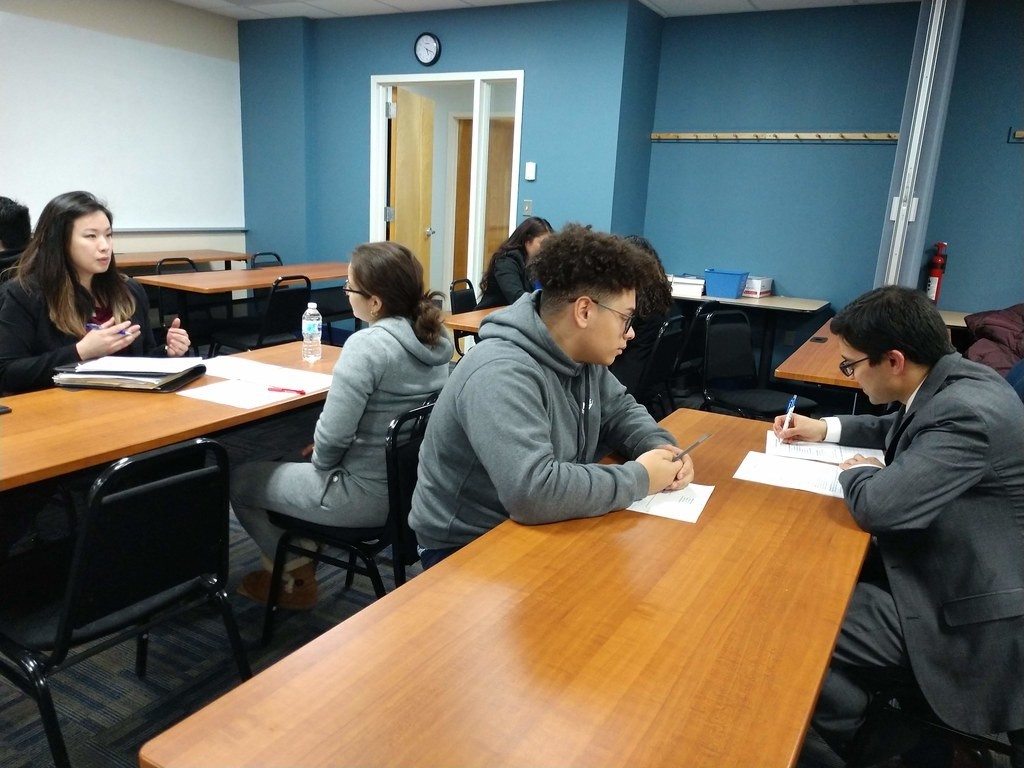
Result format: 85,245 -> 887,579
237,540 -> 319,610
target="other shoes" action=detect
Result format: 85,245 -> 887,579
952,743 -> 981,768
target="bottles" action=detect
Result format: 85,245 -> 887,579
302,302 -> 322,362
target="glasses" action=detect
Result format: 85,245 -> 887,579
839,355 -> 868,376
343,280 -> 372,300
570,297 -> 636,334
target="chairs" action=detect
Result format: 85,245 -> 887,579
846,669 -> 1024,768
1,436 -> 252,768
450,278 -> 481,368
157,252 -> 312,358
261,393 -> 440,645
637,300 -> 817,421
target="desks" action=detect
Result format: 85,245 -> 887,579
138,408 -> 872,768
0,342 -> 343,490
444,306 -> 509,332
774,317 -> 864,415
114,249 -> 253,318
938,310 -> 973,329
691,295 -> 831,388
133,263 -> 363,332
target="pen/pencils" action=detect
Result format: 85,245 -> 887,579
672,432 -> 712,461
779,395 -> 798,444
268,387 -> 305,395
86,323 -> 132,335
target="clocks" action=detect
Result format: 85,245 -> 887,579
414,32 -> 441,66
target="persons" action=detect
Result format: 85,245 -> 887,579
407,222 -> 694,572
473,217 -> 555,344
0,192 -> 191,398
772,286 -> 1024,768
228,241 -> 455,610
0,196 -> 34,281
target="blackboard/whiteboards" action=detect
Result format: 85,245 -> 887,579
0,0 -> 247,231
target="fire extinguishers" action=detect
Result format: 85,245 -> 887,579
927,242 -> 950,304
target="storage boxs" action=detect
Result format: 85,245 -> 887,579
671,269 -> 773,299
